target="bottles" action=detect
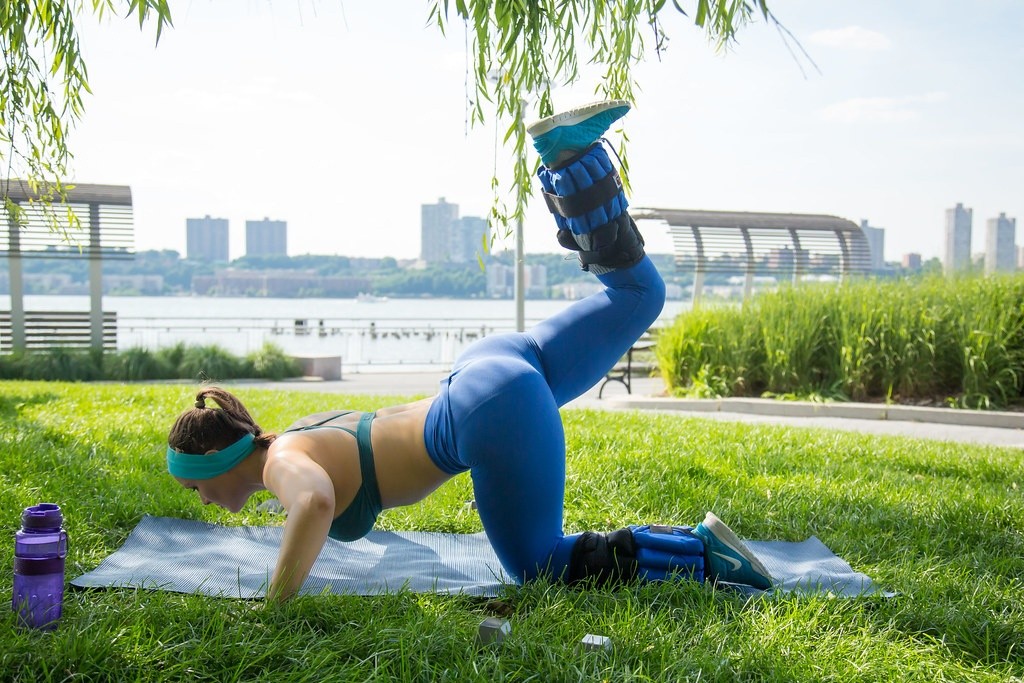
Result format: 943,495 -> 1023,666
12,503 -> 66,632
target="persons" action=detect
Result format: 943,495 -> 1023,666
167,100 -> 774,611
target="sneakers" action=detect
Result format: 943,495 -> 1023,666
527,100 -> 631,165
691,510 -> 774,592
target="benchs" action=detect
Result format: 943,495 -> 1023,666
598,330 -> 656,397
